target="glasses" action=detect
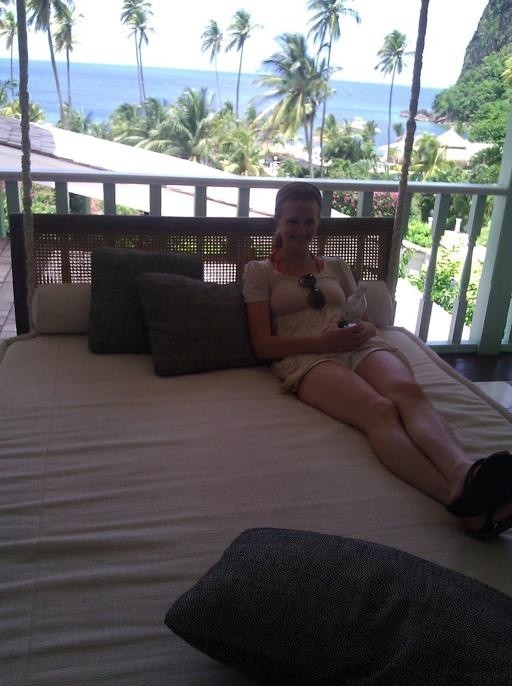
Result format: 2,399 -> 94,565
299,273 -> 325,311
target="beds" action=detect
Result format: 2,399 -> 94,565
0,213 -> 512,685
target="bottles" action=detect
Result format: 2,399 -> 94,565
337,284 -> 368,329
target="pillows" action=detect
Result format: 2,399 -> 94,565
163,527 -> 512,685
32,248 -> 261,379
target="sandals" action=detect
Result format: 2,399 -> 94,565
462,498 -> 512,538
445,452 -> 511,516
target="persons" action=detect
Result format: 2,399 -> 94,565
239,180 -> 511,540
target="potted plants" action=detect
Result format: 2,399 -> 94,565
398,221 -> 436,280
